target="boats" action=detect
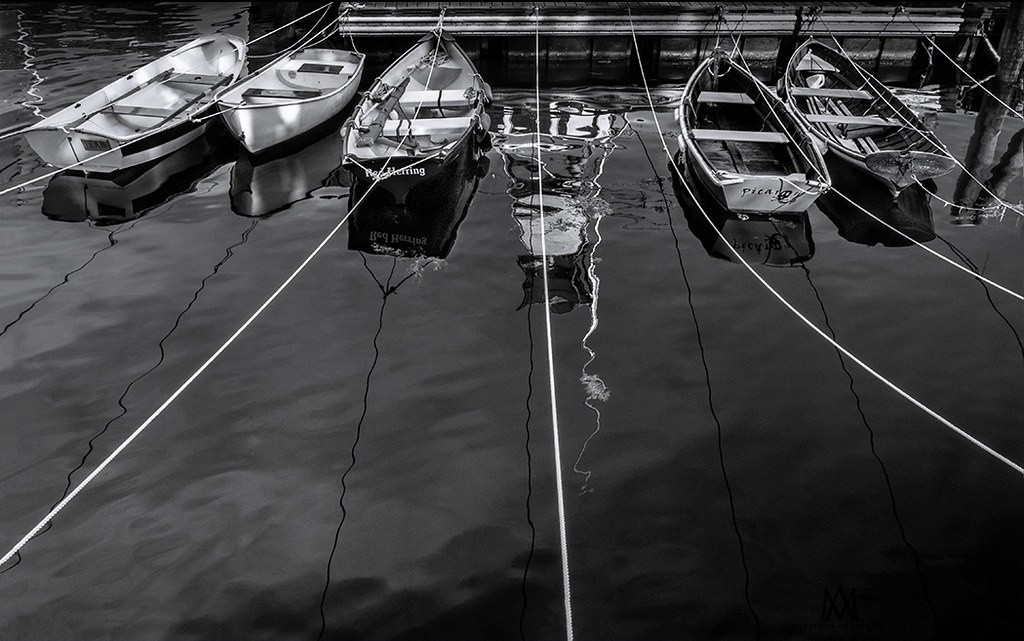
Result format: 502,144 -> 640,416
23,33 -> 246,180
41,117 -> 244,227
814,131 -> 937,248
339,23 -> 495,209
347,131 -> 487,301
674,46 -> 832,222
667,147 -> 816,268
776,35 -> 958,200
228,86 -> 367,219
213,47 -> 367,157
